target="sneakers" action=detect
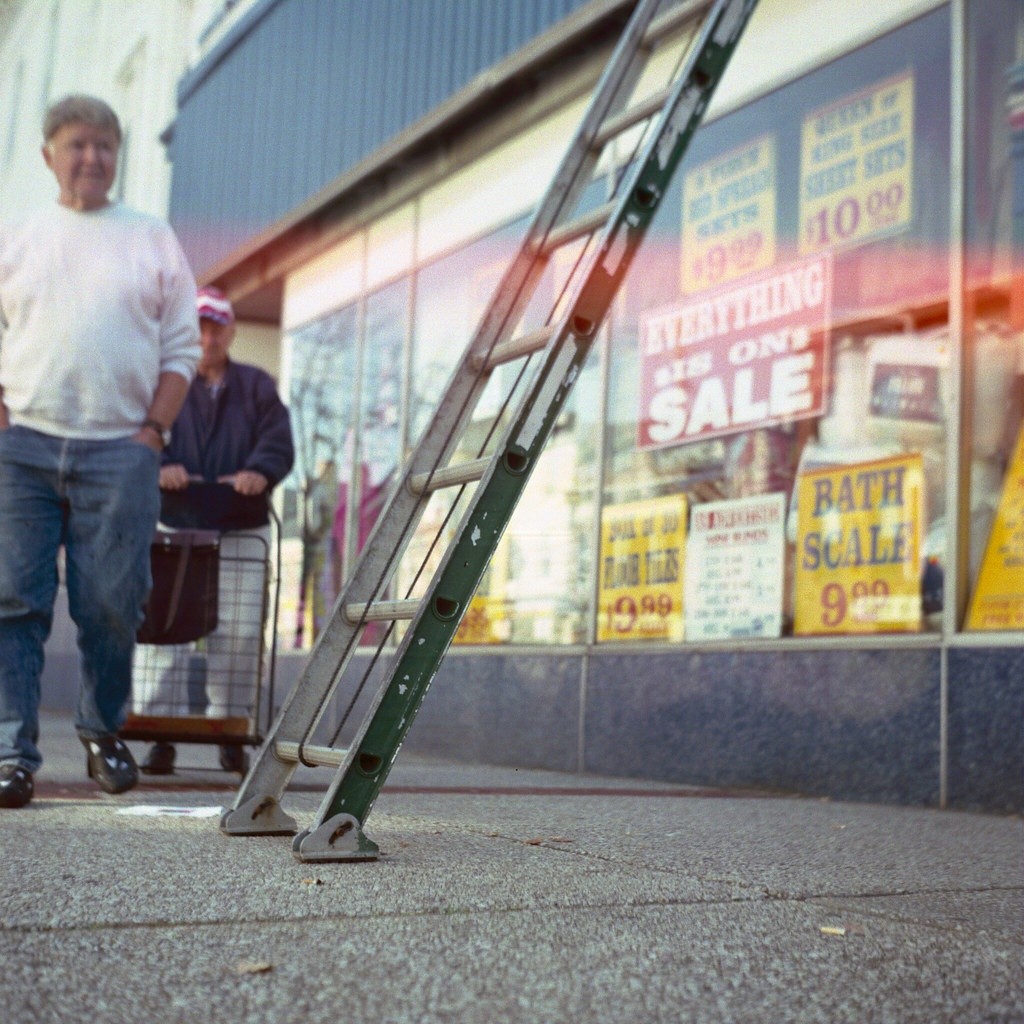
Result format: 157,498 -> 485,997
139,744 -> 176,775
217,742 -> 249,777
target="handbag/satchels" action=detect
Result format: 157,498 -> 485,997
134,527 -> 221,644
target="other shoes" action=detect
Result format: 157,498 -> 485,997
79,733 -> 138,794
0,764 -> 34,807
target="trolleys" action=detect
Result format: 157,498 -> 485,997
79,477 -> 283,777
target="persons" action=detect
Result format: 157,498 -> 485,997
134,286 -> 295,773
0,92 -> 202,807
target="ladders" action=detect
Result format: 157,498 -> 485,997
215,0 -> 757,865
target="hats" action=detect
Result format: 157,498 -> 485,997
195,284 -> 233,326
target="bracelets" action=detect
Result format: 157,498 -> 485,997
142,419 -> 165,433
